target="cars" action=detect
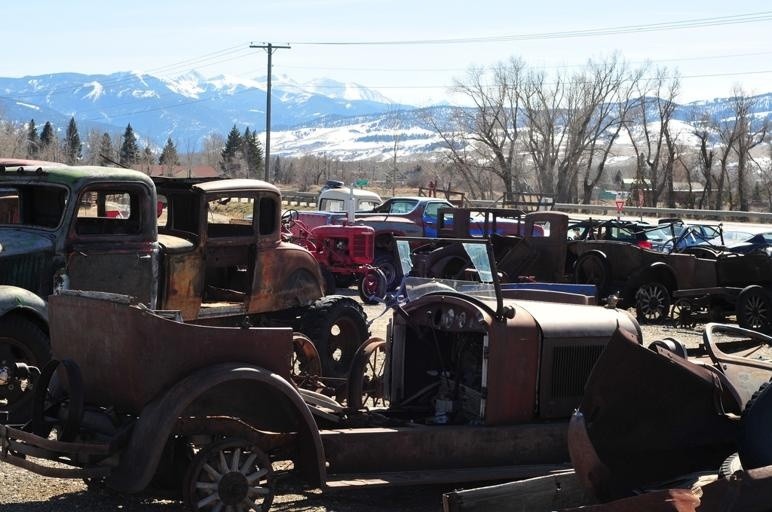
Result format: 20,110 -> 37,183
0,159 -> 771,512
